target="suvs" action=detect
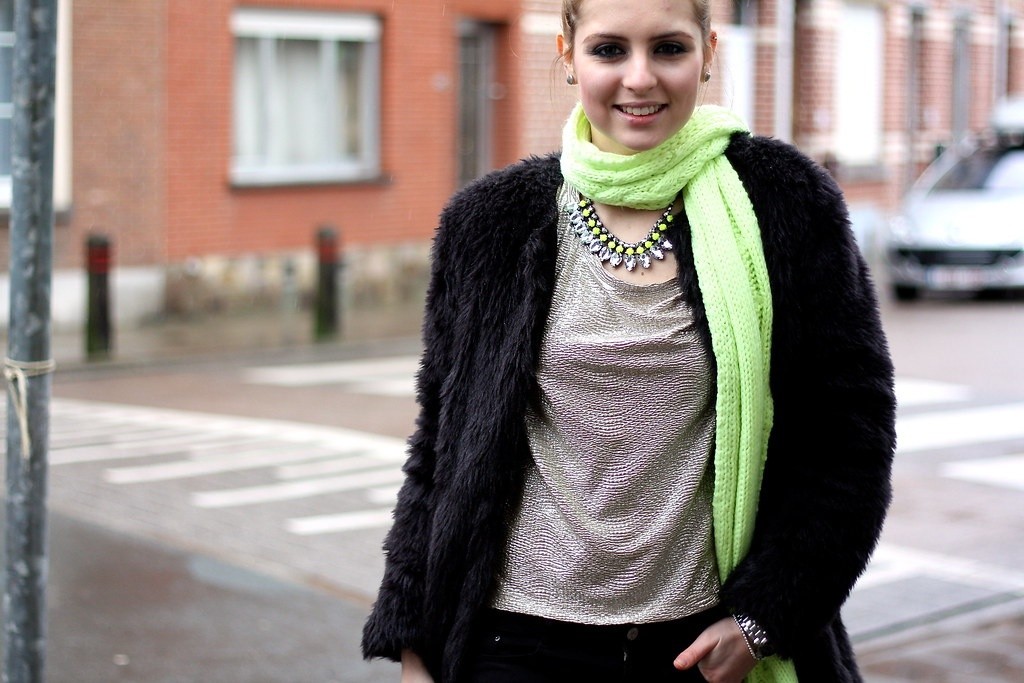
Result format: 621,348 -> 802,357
882,94 -> 1023,305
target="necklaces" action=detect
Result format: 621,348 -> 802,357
566,197 -> 674,272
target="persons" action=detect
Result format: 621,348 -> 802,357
359,0 -> 899,682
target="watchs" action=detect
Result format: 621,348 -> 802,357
731,613 -> 774,661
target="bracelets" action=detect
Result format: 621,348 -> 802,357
732,614 -> 760,662
